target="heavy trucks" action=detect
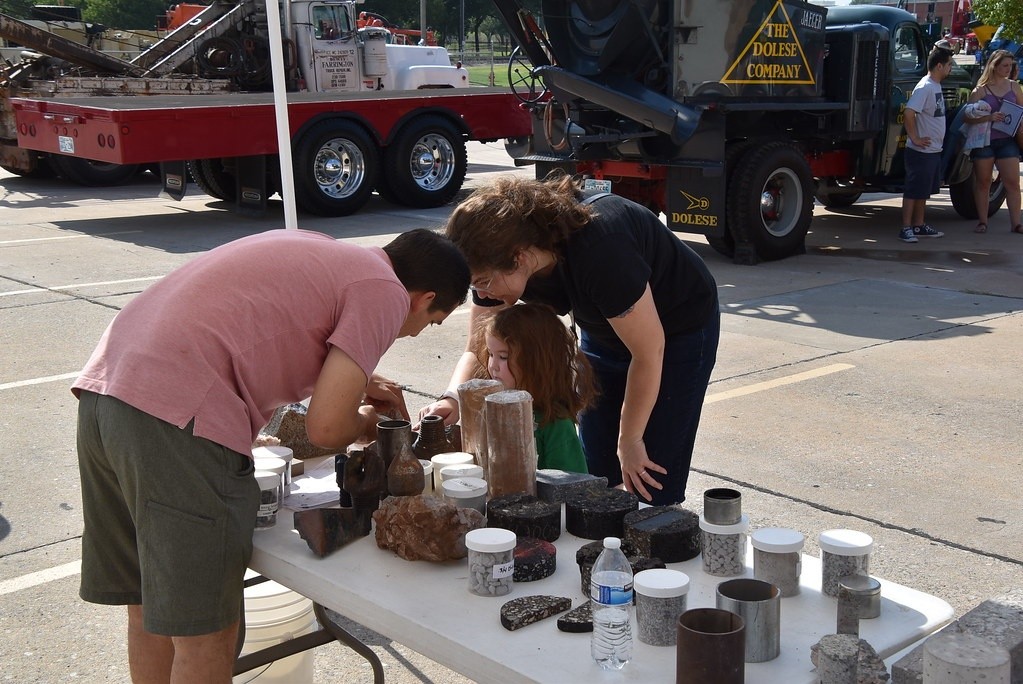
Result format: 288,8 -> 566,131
493,0 -> 1007,259
0,0 -> 470,187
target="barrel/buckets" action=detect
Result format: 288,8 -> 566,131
231,567 -> 317,684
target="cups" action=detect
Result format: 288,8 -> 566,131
676,608 -> 746,684
377,418 -> 411,501
704,488 -> 742,526
715,578 -> 780,663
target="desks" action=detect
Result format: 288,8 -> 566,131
229,450 -> 955,684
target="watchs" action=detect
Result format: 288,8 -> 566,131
435,391 -> 459,403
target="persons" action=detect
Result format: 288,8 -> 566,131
962,49 -> 1023,235
71,229 -> 474,684
411,177 -> 722,509
898,47 -> 954,243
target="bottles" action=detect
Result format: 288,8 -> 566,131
750,527 -> 804,598
590,537 -> 634,672
819,529 -> 873,601
412,416 -> 456,490
418,452 -> 516,596
699,513 -> 749,576
633,568 -> 690,647
250,445 -> 293,531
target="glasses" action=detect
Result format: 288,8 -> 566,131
469,274 -> 496,290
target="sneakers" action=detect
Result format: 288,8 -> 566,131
912,223 -> 944,237
897,226 -> 919,243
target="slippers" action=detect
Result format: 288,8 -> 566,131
1011,224 -> 1023,234
975,223 -> 988,233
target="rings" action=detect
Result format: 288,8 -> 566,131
639,470 -> 646,476
